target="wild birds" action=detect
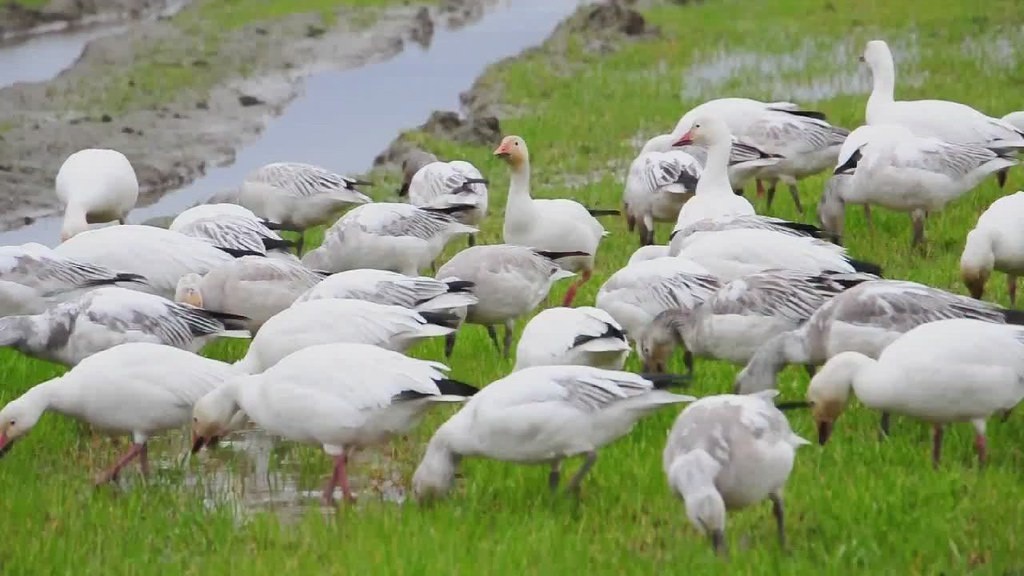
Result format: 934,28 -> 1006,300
0,121 -> 1024,353
857,40 -> 1024,154
191,298 -> 483,509
671,97 -> 851,214
0,289 -> 252,490
661,388 -> 811,557
737,279 -> 1024,474
410,308 -> 697,507
